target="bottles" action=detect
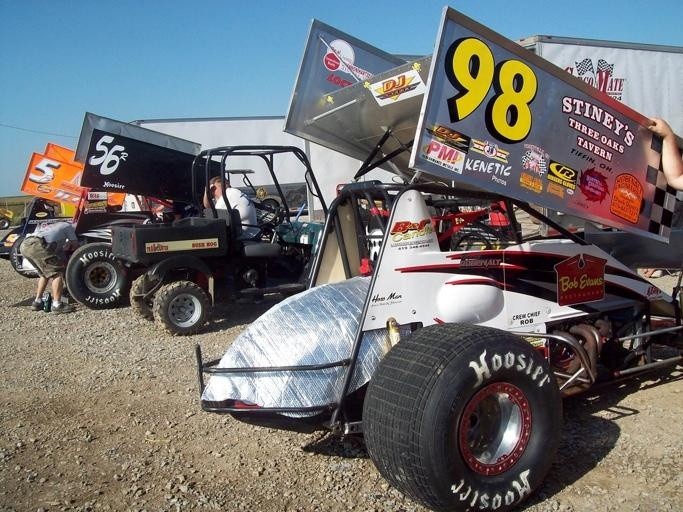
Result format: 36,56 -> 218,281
43,292 -> 52,313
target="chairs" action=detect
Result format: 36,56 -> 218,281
203,208 -> 282,259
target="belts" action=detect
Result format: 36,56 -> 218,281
25,236 -> 41,239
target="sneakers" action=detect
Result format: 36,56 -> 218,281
50,301 -> 75,314
30,299 -> 46,311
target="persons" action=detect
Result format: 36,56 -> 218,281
20,221 -> 79,315
648,114 -> 683,189
202,175 -> 257,233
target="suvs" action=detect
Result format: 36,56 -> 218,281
1,144 -> 683,512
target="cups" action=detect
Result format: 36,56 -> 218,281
303,234 -> 308,244
